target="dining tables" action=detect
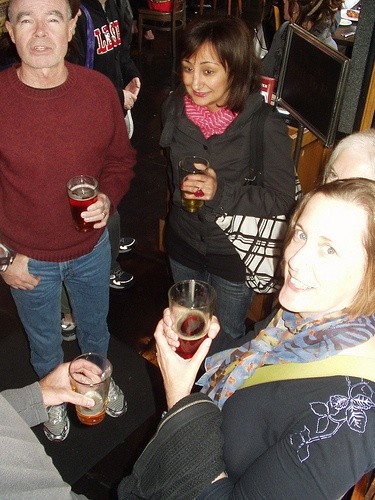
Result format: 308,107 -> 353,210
333,20 -> 357,58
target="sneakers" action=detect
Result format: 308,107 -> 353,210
104,376 -> 127,417
42,404 -> 70,442
109,266 -> 134,288
118,237 -> 136,254
61,318 -> 78,342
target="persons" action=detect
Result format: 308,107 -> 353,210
0,0 -> 375,500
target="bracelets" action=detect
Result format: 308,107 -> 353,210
0,244 -> 15,272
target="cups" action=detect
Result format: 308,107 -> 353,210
178,156 -> 211,212
168,279 -> 217,359
69,352 -> 114,425
67,175 -> 99,233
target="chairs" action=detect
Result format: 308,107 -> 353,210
137,0 -> 187,58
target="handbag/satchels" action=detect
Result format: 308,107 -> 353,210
215,104 -> 304,294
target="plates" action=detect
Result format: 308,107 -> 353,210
340,18 -> 353,26
340,8 -> 360,22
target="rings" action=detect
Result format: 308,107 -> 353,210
102,212 -> 106,216
194,189 -> 204,198
